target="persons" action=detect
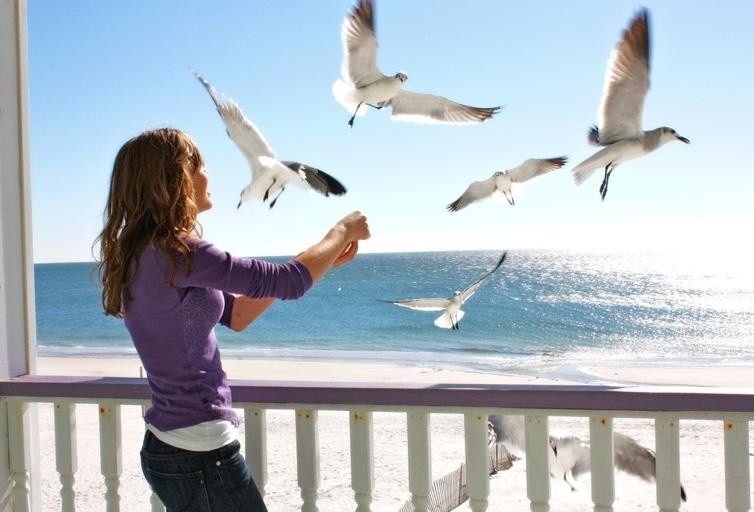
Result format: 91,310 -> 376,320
92,128 -> 370,512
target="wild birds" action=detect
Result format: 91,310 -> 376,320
448,157 -> 567,213
375,253 -> 511,334
331,1 -> 507,130
483,401 -> 688,501
573,6 -> 692,198
197,72 -> 347,212
